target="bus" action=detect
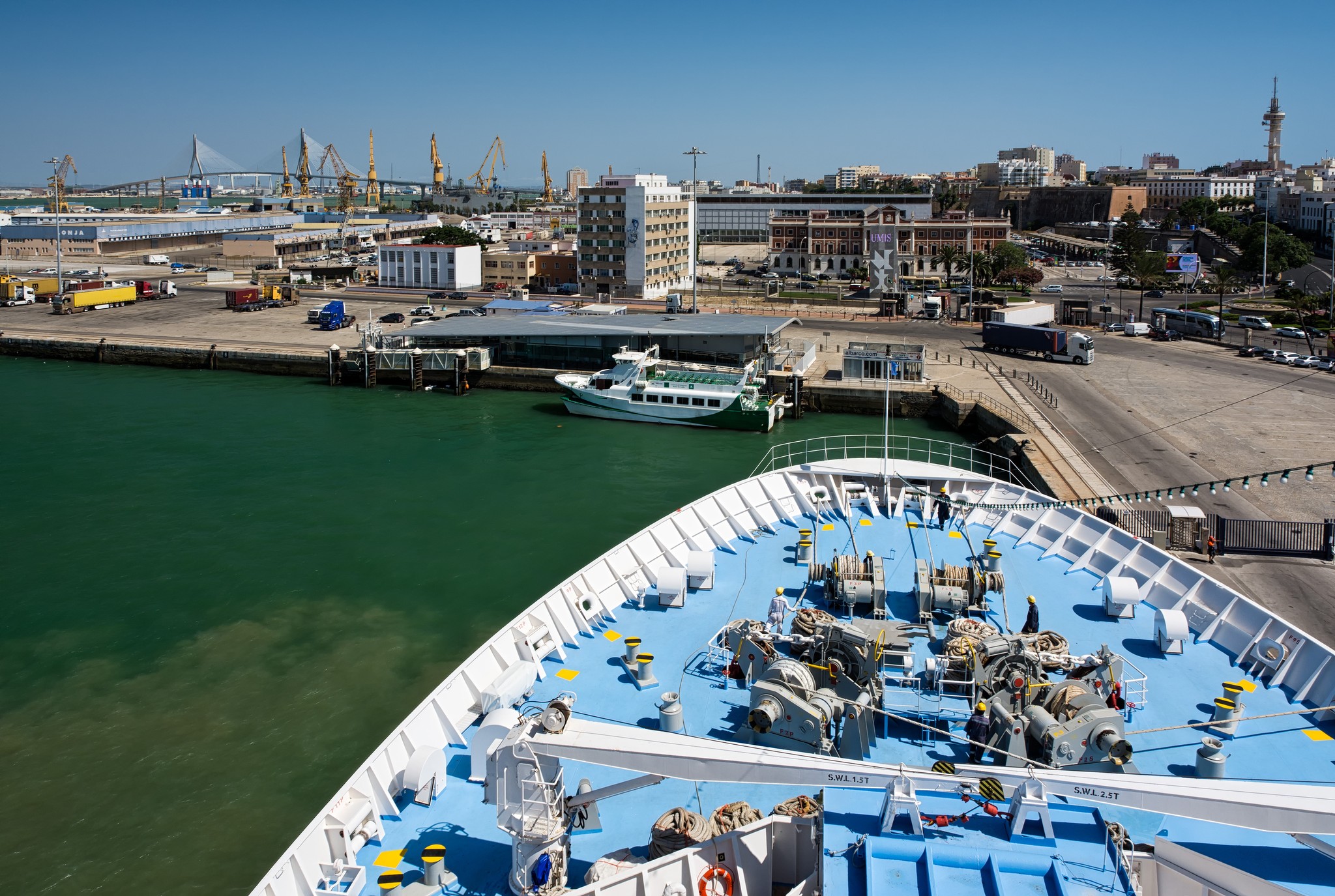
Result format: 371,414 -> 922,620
899,275 -> 942,291
1150,307 -> 1229,341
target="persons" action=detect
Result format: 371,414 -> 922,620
1208,536 -> 1216,564
1104,325 -> 1108,335
932,488 -> 951,531
1025,289 -> 1029,294
945,274 -> 946,279
505,281 -> 507,285
966,703 -> 990,764
918,295 -> 921,303
828,285 -> 830,293
1026,595 -> 1039,633
1256,283 -> 1260,291
966,277 -> 968,285
1067,272 -> 1069,277
910,293 -> 914,302
764,587 -> 796,635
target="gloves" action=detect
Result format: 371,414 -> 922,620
797,609 -> 801,611
768,614 -> 770,618
1028,626 -> 1032,633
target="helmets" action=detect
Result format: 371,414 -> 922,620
866,551 -> 875,557
775,587 -> 784,595
1209,536 -> 1213,540
977,703 -> 986,711
940,487 -> 946,492
1027,595 -> 1036,603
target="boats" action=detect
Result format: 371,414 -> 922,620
553,330 -> 794,434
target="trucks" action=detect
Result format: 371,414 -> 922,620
665,293 -> 700,314
923,292 -> 951,320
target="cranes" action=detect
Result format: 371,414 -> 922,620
47,154 -> 78,214
278,128 -> 555,214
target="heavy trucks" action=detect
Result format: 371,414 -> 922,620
143,254 -> 170,265
981,321 -> 1095,369
0,268 -> 178,315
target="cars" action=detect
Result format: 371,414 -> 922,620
1009,202 -> 1335,373
225,240 -> 577,331
171,263 -> 227,274
924,290 -> 939,298
696,258 -> 871,292
950,286 -> 976,294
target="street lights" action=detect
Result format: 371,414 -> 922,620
1093,202 -> 1101,227
0,243 -> 12,275
1262,176 -> 1290,299
758,234 -> 762,260
902,239 -> 912,292
1149,204 -> 1158,227
763,229 -> 769,256
43,157 -> 67,294
923,246 -> 928,297
1248,213 -> 1266,228
800,237 -> 807,291
682,147 -> 708,313
702,234 -> 709,290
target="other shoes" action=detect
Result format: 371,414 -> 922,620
1209,561 -> 1213,564
760,631 -> 768,634
968,757 -> 974,763
1211,560 -> 1215,563
940,526 -> 944,531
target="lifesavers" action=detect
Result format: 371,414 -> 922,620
639,368 -> 643,374
749,376 -> 752,382
699,869 -> 732,896
